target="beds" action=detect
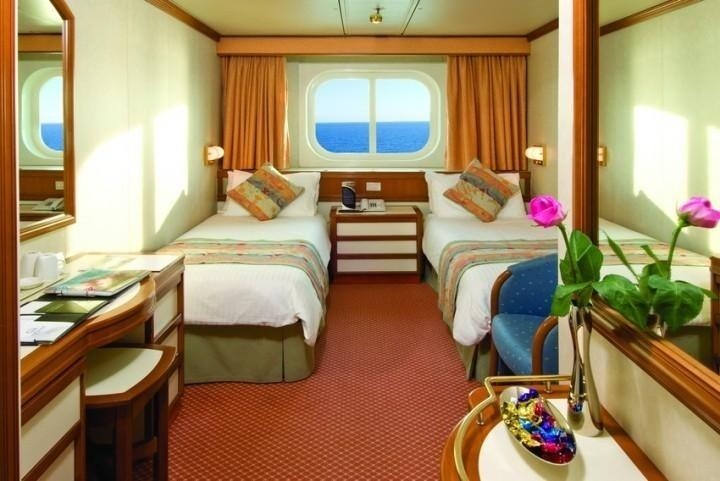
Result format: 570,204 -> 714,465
158,213 -> 332,383
598,217 -> 712,334
421,212 -> 557,385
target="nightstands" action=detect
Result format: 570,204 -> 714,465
331,205 -> 422,280
20,204 -> 65,228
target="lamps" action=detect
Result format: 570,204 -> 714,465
205,145 -> 224,166
525,146 -> 543,165
370,7 -> 382,23
599,146 -> 607,166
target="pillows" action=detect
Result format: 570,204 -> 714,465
225,167 -> 321,217
228,160 -> 305,221
444,158 -> 517,221
425,170 -> 527,216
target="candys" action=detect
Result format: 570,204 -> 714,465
502,385 -> 576,464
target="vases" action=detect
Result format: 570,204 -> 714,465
567,306 -> 603,430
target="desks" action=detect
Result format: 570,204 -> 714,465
441,377 -> 667,480
20,253 -> 185,480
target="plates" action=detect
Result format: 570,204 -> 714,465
21,276 -> 45,290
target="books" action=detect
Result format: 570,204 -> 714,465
41,266 -> 150,296
20,298 -> 109,315
20,311 -> 88,345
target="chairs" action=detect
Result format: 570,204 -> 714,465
487,252 -> 558,385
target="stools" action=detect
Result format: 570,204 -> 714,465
81,344 -> 175,480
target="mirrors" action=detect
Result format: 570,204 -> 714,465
13,0 -> 77,240
569,2 -> 720,432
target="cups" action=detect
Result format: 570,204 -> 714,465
35,255 -> 65,281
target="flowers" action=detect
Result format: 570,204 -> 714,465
528,196 -> 648,327
604,196 -> 720,334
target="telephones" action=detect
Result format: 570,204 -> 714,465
360,198 -> 385,212
